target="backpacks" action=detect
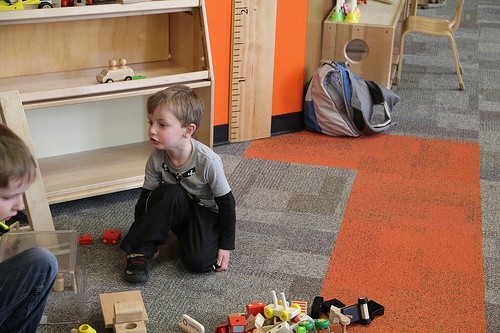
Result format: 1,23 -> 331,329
303,60 -> 400,138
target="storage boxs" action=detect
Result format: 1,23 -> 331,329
0,230 -> 89,324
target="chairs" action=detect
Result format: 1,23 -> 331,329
396,0 -> 465,90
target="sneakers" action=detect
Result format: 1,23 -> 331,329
124,252 -> 148,283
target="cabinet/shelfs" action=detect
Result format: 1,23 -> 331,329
0,0 -> 215,205
321,0 -> 410,90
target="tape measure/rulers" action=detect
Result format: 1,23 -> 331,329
228,0 -> 278,143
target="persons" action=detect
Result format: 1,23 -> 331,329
118,85 -> 237,283
0,124 -> 59,333
